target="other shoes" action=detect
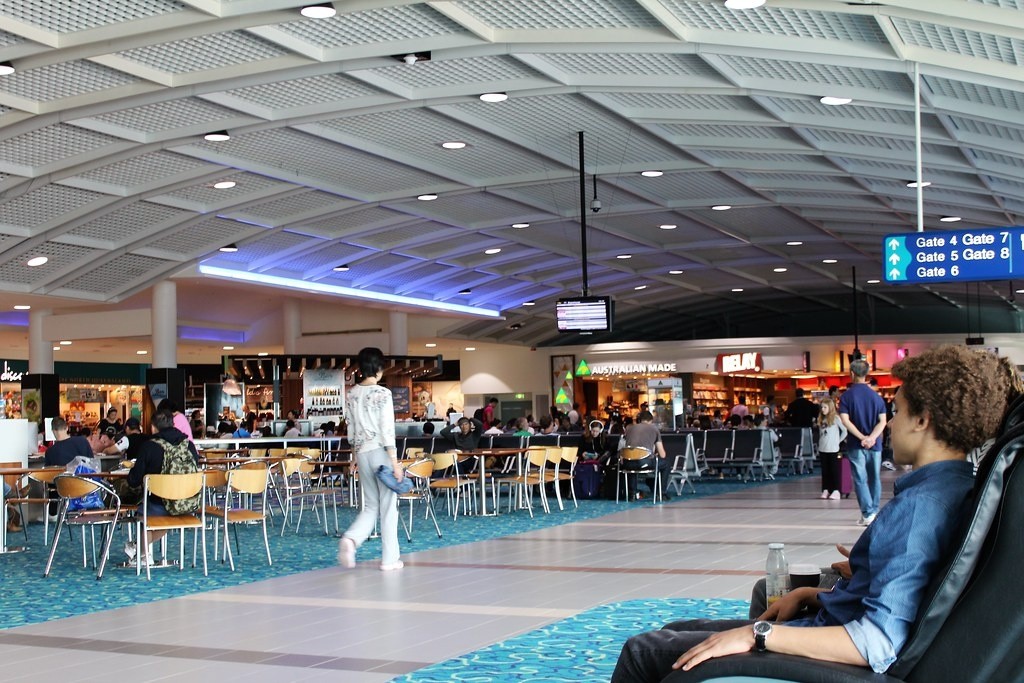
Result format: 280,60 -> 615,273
715,476 -> 724,479
9,525 -> 24,532
819,489 -> 829,499
737,474 -> 742,480
881,461 -> 896,472
338,537 -> 357,569
378,560 -> 404,571
37,513 -> 58,522
828,489 -> 841,500
708,470 -> 715,474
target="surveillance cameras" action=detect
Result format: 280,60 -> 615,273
590,200 -> 602,212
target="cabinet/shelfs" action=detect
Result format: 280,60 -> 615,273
691,373 -> 768,422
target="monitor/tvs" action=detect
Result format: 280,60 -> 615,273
555,296 -> 612,333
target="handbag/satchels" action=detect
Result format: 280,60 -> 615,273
374,464 -> 415,496
61,455 -> 106,511
770,447 -> 781,474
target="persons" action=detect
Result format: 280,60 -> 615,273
711,396 -> 784,480
439,398 -> 535,498
422,422 -> 435,437
611,345 -> 1010,683
535,404 -> 632,498
829,386 -> 839,408
966,357 -> 1024,478
817,399 -> 847,499
623,410 -> 671,500
191,405 -> 301,439
314,421 -> 348,450
783,388 -> 820,473
839,359 -> 887,526
37,399 -> 203,565
338,347 -> 404,571
882,385 -> 908,471
693,420 -> 702,431
5,483 -> 32,531
641,403 -> 646,411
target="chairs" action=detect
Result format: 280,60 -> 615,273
660,384 -> 1024,682
616,445 -> 663,506
0,444 -> 579,580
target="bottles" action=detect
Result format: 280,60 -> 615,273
308,407 -> 343,415
766,543 -> 791,611
312,397 -> 340,405
309,388 -> 340,395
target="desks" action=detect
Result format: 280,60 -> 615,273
475,449 -> 547,508
319,448 -> 358,508
79,471 -> 179,567
198,456 -> 307,526
0,468 -> 64,555
198,448 -> 250,499
458,451 -> 517,517
310,460 -> 382,538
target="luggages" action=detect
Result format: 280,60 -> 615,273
821,456 -> 852,498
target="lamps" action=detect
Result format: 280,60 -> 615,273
835,351 -> 843,373
802,351 -> 811,372
865,349 -> 875,372
897,348 -> 909,360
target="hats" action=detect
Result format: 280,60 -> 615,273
699,405 -> 706,409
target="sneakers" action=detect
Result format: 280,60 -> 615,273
127,554 -> 155,567
854,512 -> 875,527
123,538 -> 138,560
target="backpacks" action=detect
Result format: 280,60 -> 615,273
572,463 -> 599,499
148,435 -> 202,516
473,405 -> 490,425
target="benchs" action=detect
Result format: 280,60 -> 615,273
398,425 -> 818,496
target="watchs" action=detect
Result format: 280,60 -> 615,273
753,621 -> 772,652
